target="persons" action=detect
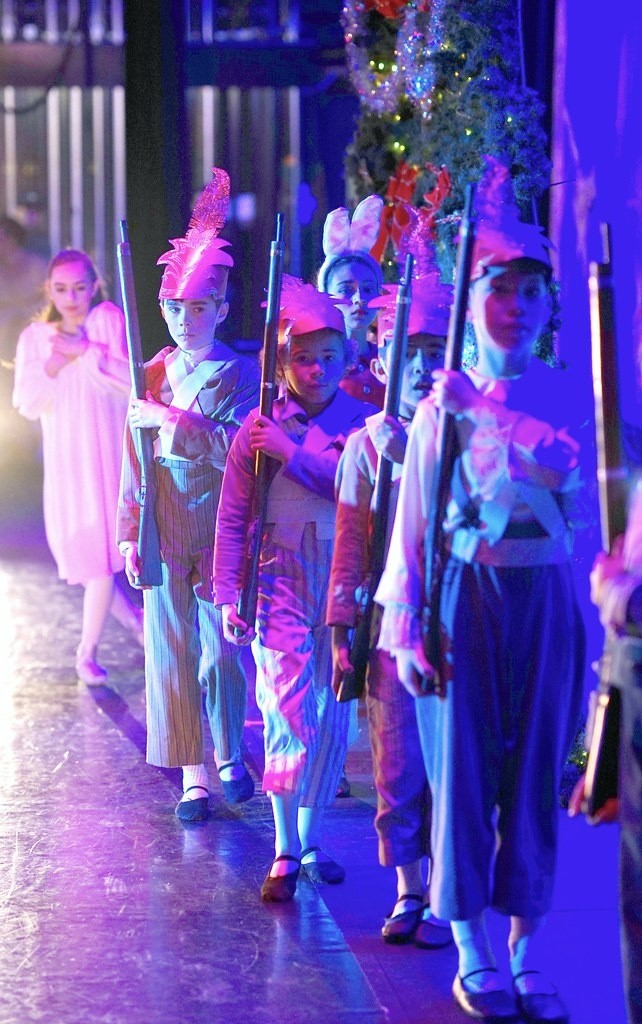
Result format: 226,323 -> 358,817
327,313 -> 479,951
371,232 -> 586,1024
12,248 -> 147,686
115,237 -> 265,822
211,276 -> 386,908
0,215 -> 56,499
564,477 -> 640,1024
313,250 -> 386,378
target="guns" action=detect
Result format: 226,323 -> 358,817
331,251 -> 417,705
582,215 -> 627,822
230,210 -> 286,640
417,178 -> 482,702
114,216 -> 168,590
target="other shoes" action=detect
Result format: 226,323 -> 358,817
75,642 -> 108,685
511,970 -> 570,1024
380,893 -> 423,942
260,855 -> 301,903
300,846 -> 346,884
175,786 -> 210,822
415,903 -> 453,948
218,761 -> 255,804
451,968 -> 519,1020
